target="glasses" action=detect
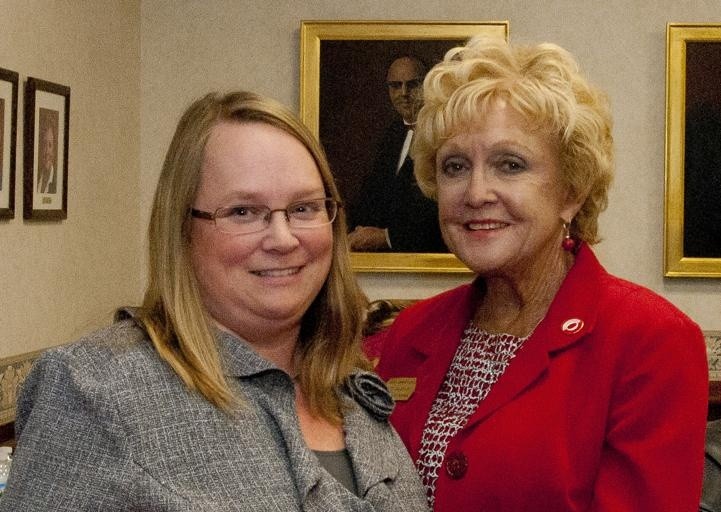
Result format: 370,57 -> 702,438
187,194 -> 341,234
384,79 -> 420,90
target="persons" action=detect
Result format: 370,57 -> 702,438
0,91 -> 431,511
348,54 -> 455,252
38,110 -> 58,195
372,33 -> 709,511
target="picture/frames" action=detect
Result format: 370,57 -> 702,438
0,66 -> 18,221
297,15 -> 510,278
22,73 -> 70,221
659,19 -> 721,282
